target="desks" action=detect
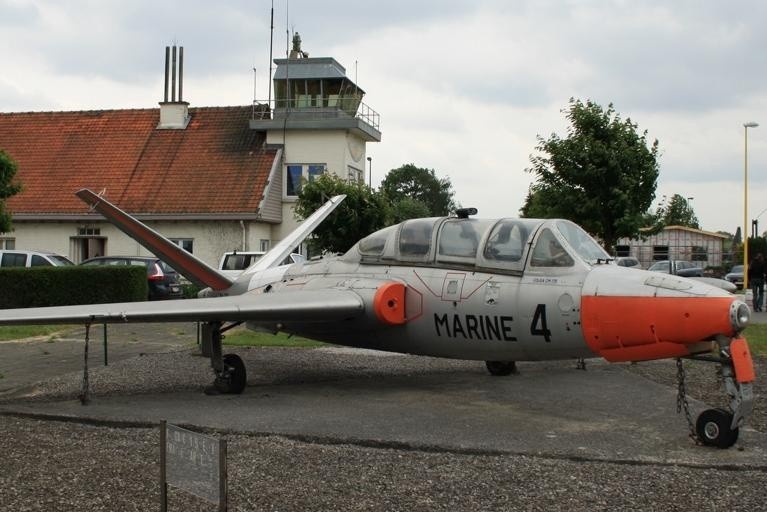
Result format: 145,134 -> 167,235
366,156 -> 371,189
743,121 -> 758,285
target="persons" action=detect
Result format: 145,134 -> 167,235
747,252 -> 765,312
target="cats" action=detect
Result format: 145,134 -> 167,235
1,189 -> 755,449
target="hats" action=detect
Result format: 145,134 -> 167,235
647,260 -> 702,277
726,265 -> 745,288
614,256 -> 642,269
2,249 -> 185,300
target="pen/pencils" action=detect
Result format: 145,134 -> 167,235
218,251 -> 306,286
